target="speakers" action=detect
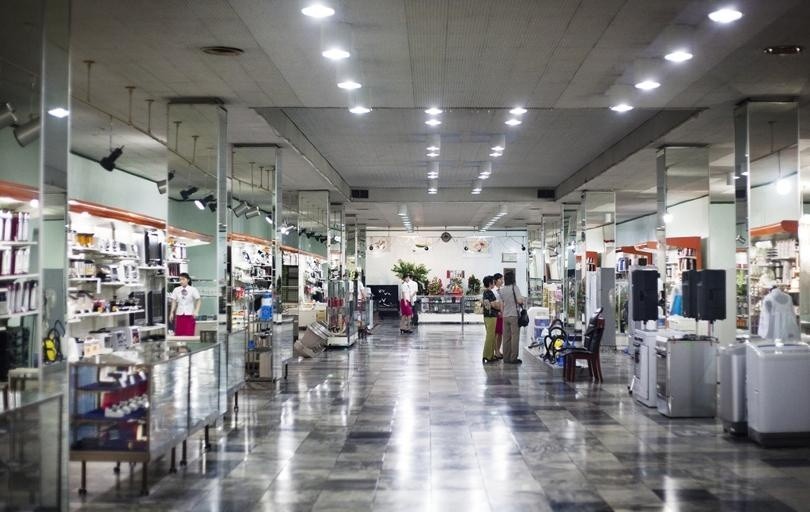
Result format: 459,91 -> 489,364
537,189 -> 555,198
632,270 -> 659,321
352,189 -> 368,199
682,271 -> 698,317
695,268 -> 726,320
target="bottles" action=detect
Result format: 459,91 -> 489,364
0,246 -> 29,275
6,280 -> 38,313
0,208 -> 30,241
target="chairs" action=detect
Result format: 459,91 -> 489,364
563,317 -> 607,384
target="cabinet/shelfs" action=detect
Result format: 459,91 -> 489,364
575,220 -> 799,334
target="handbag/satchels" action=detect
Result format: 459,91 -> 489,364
518,309 -> 529,328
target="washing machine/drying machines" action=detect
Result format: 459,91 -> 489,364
745,337 -> 810,446
719,344 -> 750,436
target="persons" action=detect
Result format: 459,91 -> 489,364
354,272 -> 366,310
169,273 -> 201,336
491,273 -> 503,359
483,276 -> 500,362
757,288 -> 800,341
500,272 -> 522,364
400,274 -> 418,333
